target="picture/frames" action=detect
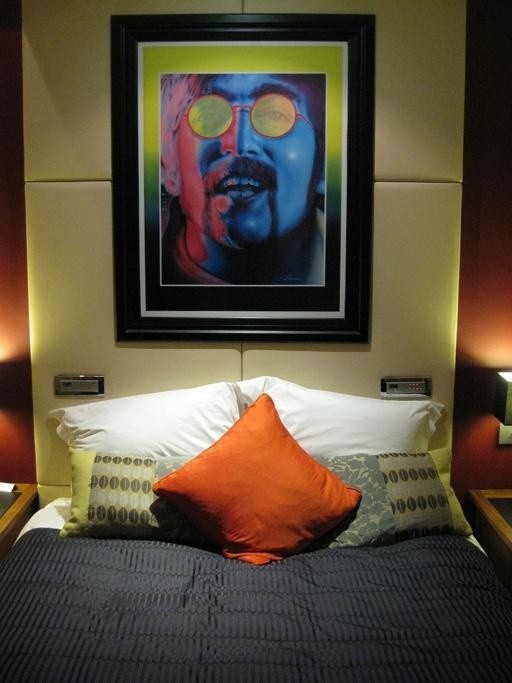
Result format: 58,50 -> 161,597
109,14 -> 376,344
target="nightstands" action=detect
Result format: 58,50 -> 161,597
466,488 -> 512,591
0,482 -> 38,564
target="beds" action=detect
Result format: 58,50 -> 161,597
0,496 -> 512,683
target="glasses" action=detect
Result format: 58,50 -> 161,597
184,93 -> 312,140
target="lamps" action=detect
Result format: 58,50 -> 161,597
494,371 -> 512,425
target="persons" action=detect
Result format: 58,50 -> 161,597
159,72 -> 325,285
